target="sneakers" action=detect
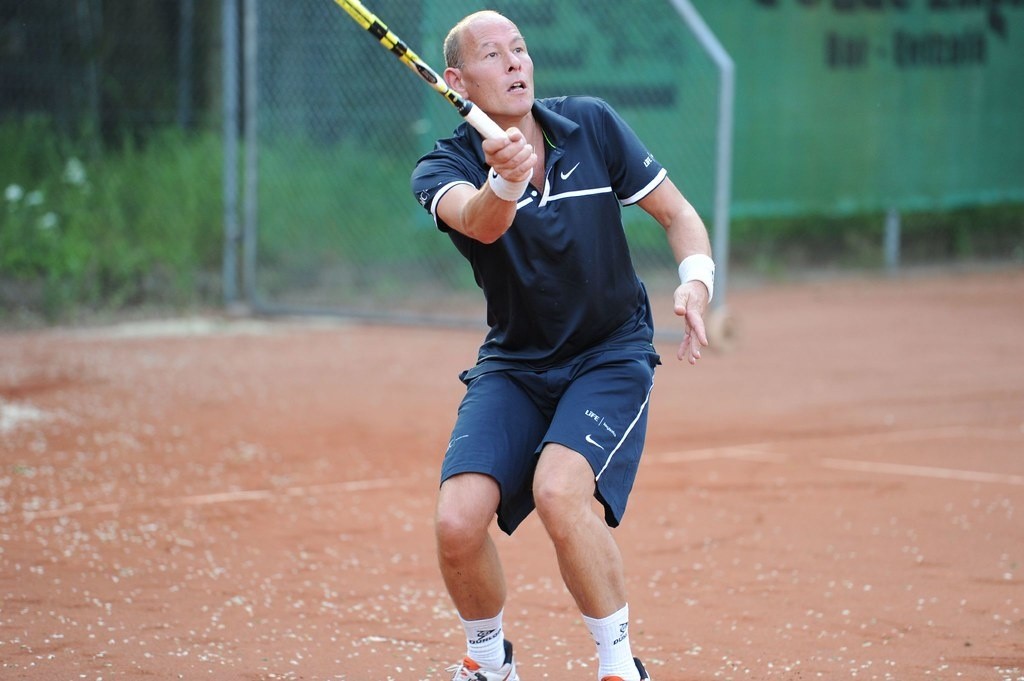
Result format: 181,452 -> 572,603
599,656 -> 650,681
451,638 -> 520,681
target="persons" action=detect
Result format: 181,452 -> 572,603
411,10 -> 714,681
